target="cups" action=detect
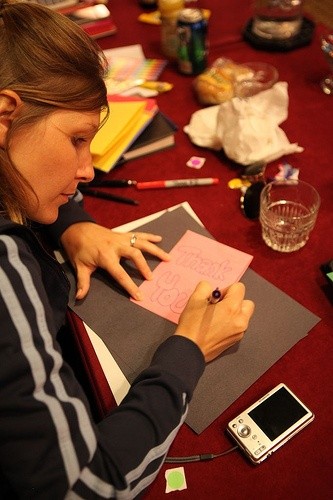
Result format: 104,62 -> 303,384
260,179 -> 320,252
252,0 -> 303,40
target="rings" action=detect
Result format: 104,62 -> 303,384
130,235 -> 136,244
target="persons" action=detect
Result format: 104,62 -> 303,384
0,2 -> 255,500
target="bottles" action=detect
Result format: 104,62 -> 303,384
158,0 -> 184,57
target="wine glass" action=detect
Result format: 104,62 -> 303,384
320,30 -> 333,98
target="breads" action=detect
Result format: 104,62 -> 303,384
194,68 -> 234,106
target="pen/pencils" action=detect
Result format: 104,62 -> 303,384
137,178 -> 219,190
140,81 -> 173,92
77,180 -> 139,206
209,290 -> 220,304
139,14 -> 160,25
321,35 -> 333,95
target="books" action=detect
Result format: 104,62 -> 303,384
121,112 -> 175,162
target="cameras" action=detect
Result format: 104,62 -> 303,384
226,382 -> 315,465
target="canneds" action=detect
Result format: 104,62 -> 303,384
175,8 -> 206,75
